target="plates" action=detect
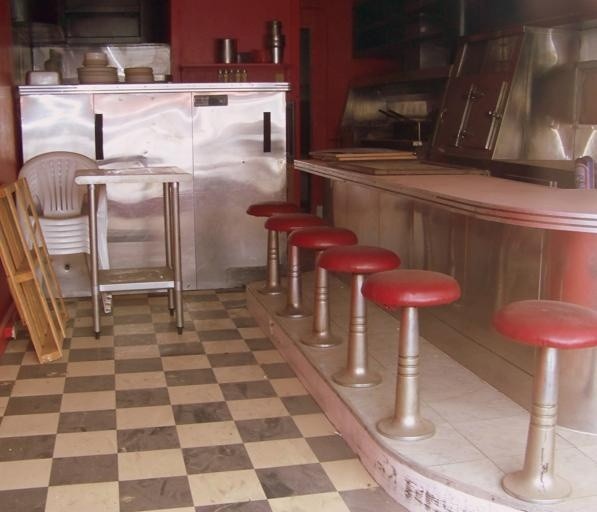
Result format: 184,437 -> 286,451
77,67 -> 118,83
29,72 -> 59,85
124,68 -> 153,82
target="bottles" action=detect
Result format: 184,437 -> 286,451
218,70 -> 248,81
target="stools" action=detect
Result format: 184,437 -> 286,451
491,299 -> 596,503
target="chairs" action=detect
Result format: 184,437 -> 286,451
16,151 -> 112,314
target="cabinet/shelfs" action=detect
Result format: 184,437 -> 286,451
74,168 -> 191,340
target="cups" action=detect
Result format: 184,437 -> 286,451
214,37 -> 236,64
265,21 -> 281,36
265,35 -> 285,64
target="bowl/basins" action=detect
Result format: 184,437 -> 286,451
82,53 -> 108,68
238,53 -> 260,63
